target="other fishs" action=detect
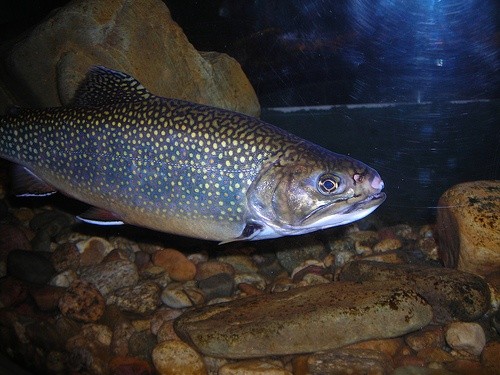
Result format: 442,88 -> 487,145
0,66 -> 388,247
